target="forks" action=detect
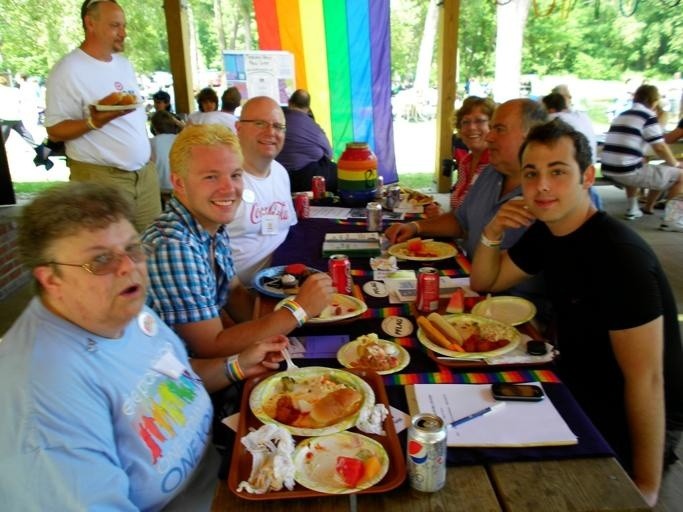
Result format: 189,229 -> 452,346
279,347 -> 304,379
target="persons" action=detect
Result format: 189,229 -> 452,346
371,98 -> 606,301
42,0 -> 160,236
447,93 -> 501,208
0,179 -> 294,512
151,91 -> 180,122
596,84 -> 682,234
270,89 -> 338,192
653,91 -> 683,210
547,84 -> 598,148
653,103 -> 667,127
184,89 -> 220,125
467,117 -> 681,511
216,96 -> 302,288
129,123 -> 335,420
541,92 -> 604,212
194,87 -> 241,131
145,111 -> 181,208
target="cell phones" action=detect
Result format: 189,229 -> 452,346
491,381 -> 545,402
527,339 -> 547,356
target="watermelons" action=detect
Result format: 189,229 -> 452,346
446,288 -> 464,314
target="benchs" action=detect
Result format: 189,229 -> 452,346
593,177 -> 618,185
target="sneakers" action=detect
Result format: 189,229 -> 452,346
625,208 -> 643,220
660,217 -> 682,231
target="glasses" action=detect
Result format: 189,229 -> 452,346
239,119 -> 287,132
48,241 -> 148,277
459,116 -> 490,128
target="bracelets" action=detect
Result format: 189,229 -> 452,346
408,219 -> 422,235
277,299 -> 309,329
81,106 -> 100,132
222,350 -> 243,385
478,232 -> 505,248
673,161 -> 680,168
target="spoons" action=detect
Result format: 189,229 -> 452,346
479,293 -> 494,318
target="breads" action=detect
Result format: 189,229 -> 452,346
310,388 -> 364,421
99,91 -> 136,105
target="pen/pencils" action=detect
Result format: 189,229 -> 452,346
445,401 -> 505,428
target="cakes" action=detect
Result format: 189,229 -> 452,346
280,274 -> 299,293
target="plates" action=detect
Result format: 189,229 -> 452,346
416,313 -> 522,359
380,193 -> 434,210
470,295 -> 536,328
336,337 -> 411,376
273,290 -> 370,326
387,239 -> 460,262
288,428 -> 389,494
249,265 -> 321,299
92,99 -> 144,112
248,365 -> 376,438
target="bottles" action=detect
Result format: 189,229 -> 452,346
336,142 -> 378,202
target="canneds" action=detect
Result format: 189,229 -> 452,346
407,413 -> 447,493
367,202 -> 383,232
328,255 -> 352,296
295,192 -> 310,218
416,266 -> 440,313
313,176 -> 326,198
387,186 -> 400,208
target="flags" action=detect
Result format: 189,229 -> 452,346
251,0 -> 399,186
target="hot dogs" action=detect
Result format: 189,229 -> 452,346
417,312 -> 464,350
